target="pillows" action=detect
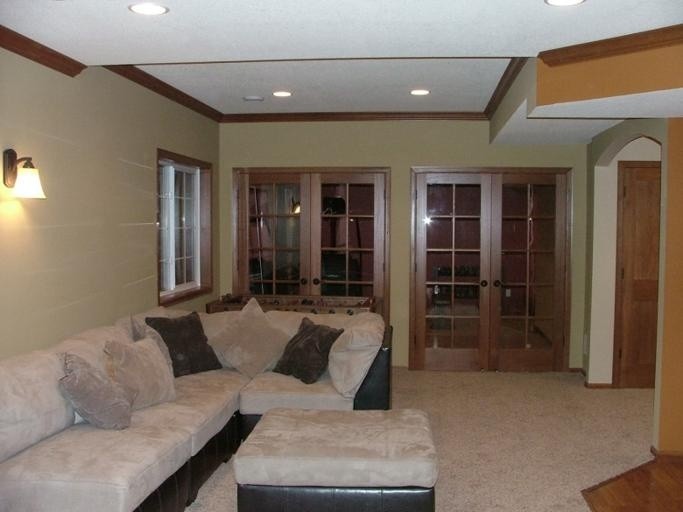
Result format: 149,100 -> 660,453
272,316 -> 343,384
62,352 -> 136,428
207,296 -> 291,379
144,310 -> 223,376
329,312 -> 385,399
105,335 -> 175,410
130,312 -> 174,377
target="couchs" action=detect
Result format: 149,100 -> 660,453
1,307 -> 391,512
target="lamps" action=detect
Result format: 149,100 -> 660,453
3,148 -> 47,201
289,196 -> 302,215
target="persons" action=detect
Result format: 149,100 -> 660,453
334,198 -> 360,267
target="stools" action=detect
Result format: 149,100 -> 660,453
232,407 -> 438,512
